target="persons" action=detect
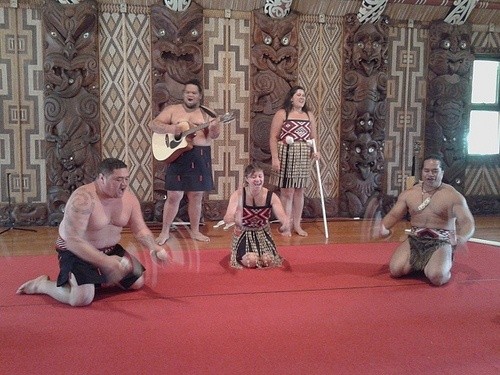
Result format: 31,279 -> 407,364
148,79 -> 222,246
223,163 -> 291,269
382,156 -> 476,287
16,158 -> 169,306
270,85 -> 320,237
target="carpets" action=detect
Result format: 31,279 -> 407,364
0,241 -> 500,375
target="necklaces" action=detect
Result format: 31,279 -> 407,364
249,187 -> 258,210
417,183 -> 442,211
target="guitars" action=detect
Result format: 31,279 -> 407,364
151,110 -> 236,164
